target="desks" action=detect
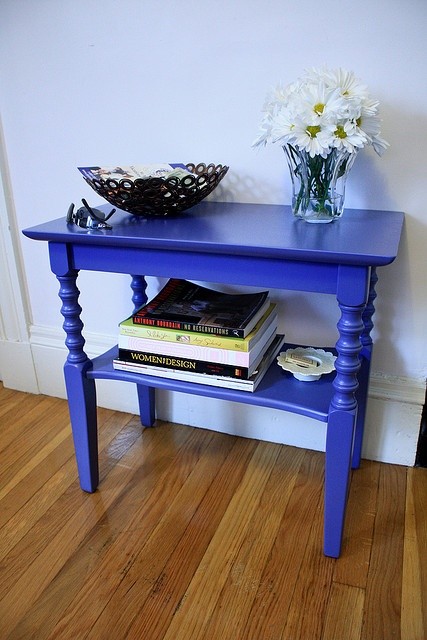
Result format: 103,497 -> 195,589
21,203 -> 405,559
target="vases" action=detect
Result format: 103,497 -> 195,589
281,144 -> 360,223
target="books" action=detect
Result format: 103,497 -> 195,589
111,277 -> 288,394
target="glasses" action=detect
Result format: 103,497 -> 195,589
66,198 -> 116,230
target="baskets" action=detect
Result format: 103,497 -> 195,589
77,162 -> 229,217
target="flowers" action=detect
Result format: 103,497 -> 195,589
250,65 -> 390,218
